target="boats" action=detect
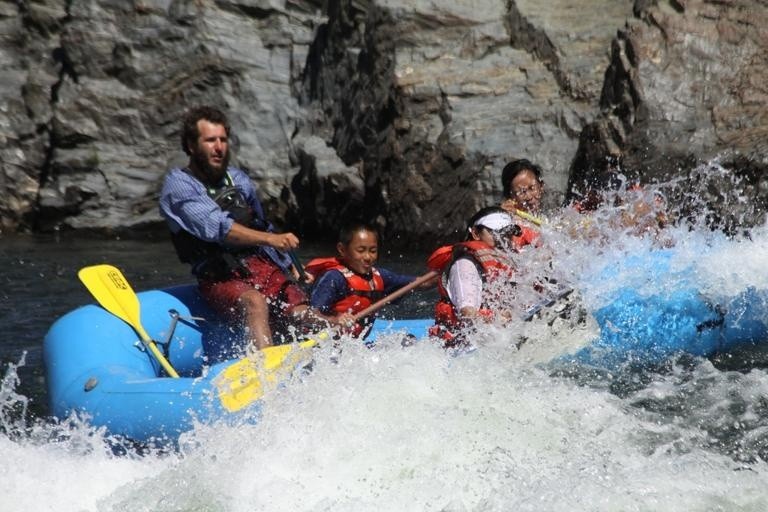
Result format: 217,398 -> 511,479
42,250 -> 767,452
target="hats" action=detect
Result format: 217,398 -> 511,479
466,207 -> 522,236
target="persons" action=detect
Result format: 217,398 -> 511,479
303,216 -> 438,351
427,159 -> 675,357
156,104 -> 320,354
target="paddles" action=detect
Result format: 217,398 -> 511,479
77,264 -> 180,378
209,271 -> 439,413
